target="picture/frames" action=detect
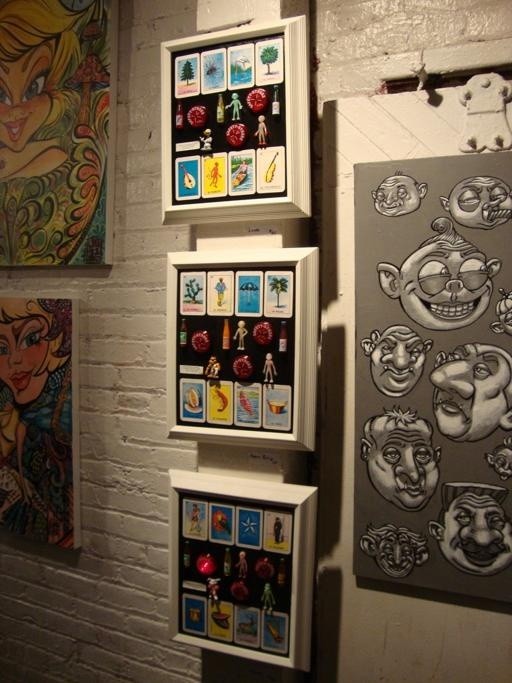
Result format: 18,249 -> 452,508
169,468 -> 320,675
165,246 -> 319,452
160,14 -> 311,225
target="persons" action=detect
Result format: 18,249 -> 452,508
1,0 -> 112,270
0,298 -> 73,549
